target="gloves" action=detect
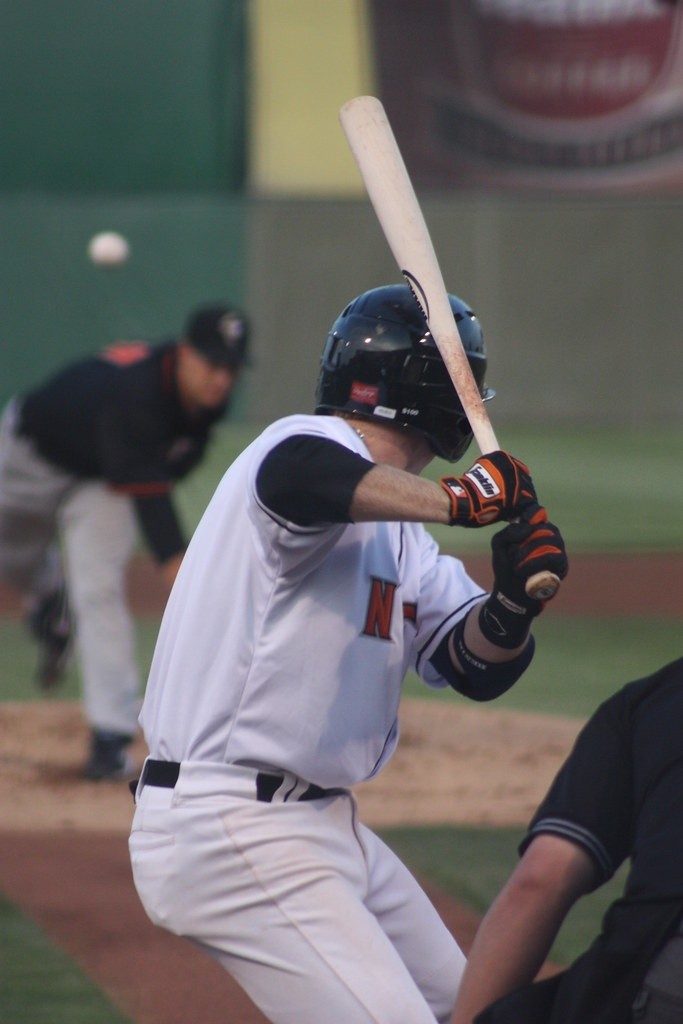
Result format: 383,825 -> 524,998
440,451 -> 539,527
486,507 -> 569,619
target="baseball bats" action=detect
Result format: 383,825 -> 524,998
338,96 -> 562,604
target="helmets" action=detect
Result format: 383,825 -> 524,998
313,284 -> 495,462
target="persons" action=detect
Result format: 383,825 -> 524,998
451,658 -> 683,1024
1,300 -> 251,783
129,283 -> 568,1024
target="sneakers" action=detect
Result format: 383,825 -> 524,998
79,734 -> 138,781
27,599 -> 72,693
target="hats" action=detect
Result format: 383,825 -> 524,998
176,302 -> 257,373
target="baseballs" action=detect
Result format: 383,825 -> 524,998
89,231 -> 130,268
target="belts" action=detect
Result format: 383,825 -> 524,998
128,758 -> 343,803
